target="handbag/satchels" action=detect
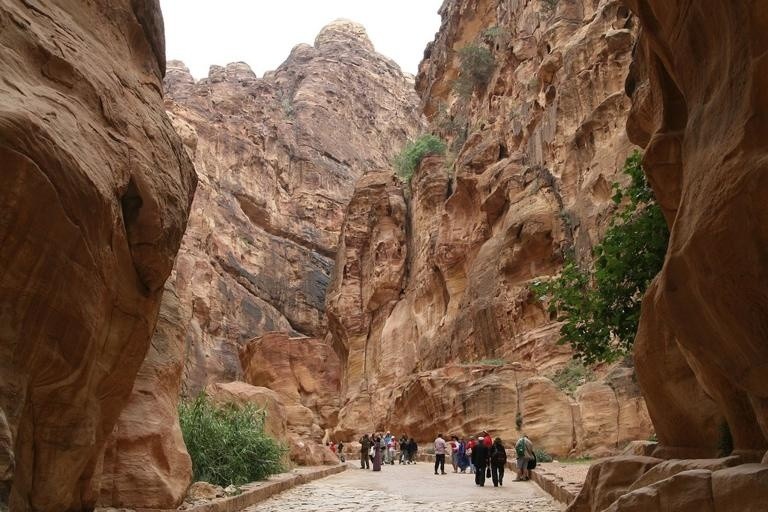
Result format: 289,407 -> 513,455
527,451 -> 536,470
486,465 -> 491,478
465,442 -> 472,456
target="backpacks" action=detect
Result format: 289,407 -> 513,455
492,445 -> 505,464
515,438 -> 525,457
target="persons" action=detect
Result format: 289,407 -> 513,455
325,429 -> 536,489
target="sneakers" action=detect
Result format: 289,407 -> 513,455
511,475 -> 529,482
360,461 -> 417,471
434,470 -> 476,475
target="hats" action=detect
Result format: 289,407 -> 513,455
478,436 -> 485,441
391,436 -> 395,439
